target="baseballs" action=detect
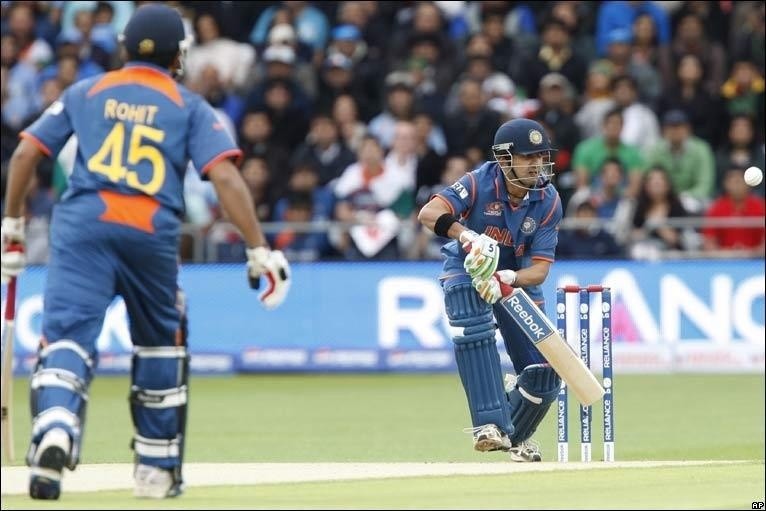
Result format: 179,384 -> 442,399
745,167 -> 764,187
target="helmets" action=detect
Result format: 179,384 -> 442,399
491,118 -> 558,190
118,5 -> 188,81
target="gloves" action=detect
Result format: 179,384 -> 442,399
460,230 -> 516,304
246,248 -> 290,307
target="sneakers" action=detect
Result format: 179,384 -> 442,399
473,423 -> 541,462
30,427 -> 71,499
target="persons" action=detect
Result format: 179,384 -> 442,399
1,0 -> 766,260
416,118 -> 563,463
1,4 -> 291,502
